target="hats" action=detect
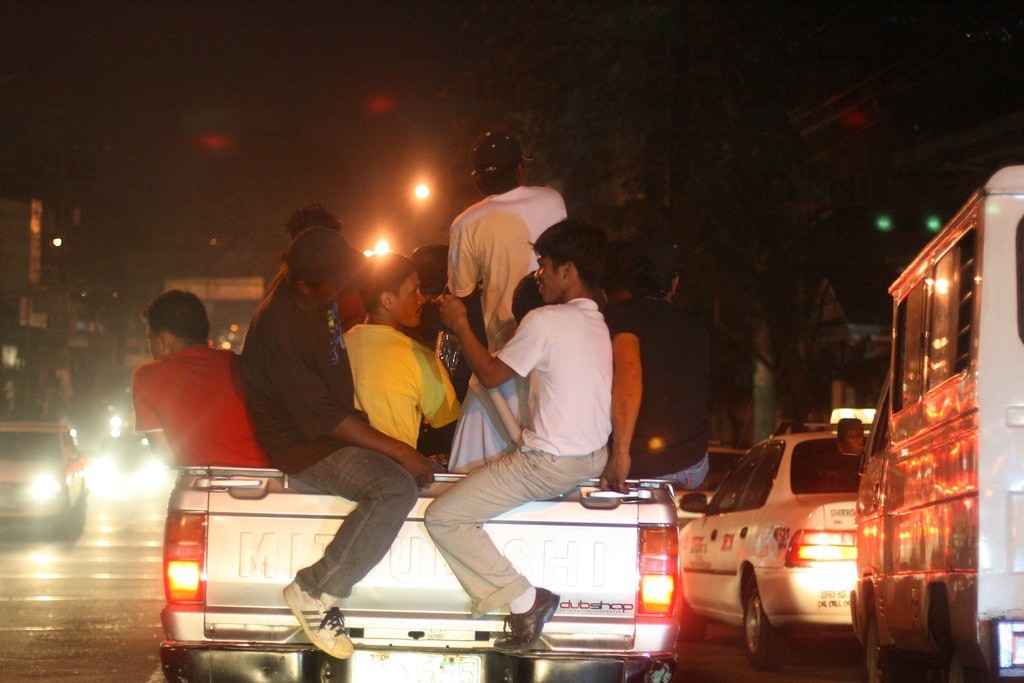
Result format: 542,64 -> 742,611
280,226 -> 365,287
469,131 -> 536,176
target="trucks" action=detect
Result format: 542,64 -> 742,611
856,165 -> 1024,683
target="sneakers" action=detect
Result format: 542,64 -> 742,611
284,580 -> 355,659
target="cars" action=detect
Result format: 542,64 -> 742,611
680,430 -> 870,669
671,448 -> 748,526
0,422 -> 89,540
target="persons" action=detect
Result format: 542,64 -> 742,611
838,419 -> 864,454
131,127 -> 715,659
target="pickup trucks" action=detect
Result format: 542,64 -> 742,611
155,459 -> 679,683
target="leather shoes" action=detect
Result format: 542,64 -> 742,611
494,587 -> 560,654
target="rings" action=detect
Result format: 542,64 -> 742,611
617,483 -> 625,489
